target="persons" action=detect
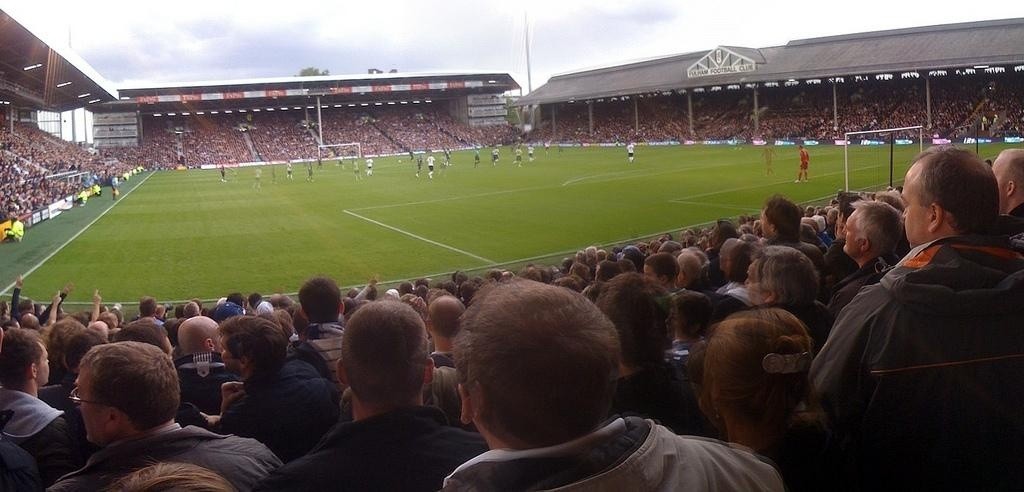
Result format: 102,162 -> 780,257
0,118 -> 145,243
140,103 -> 526,171
263,144 -> 1023,491
220,165 -> 227,183
0,272 -> 346,492
518,72 -> 1024,145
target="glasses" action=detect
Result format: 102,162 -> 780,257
68,387 -> 109,407
